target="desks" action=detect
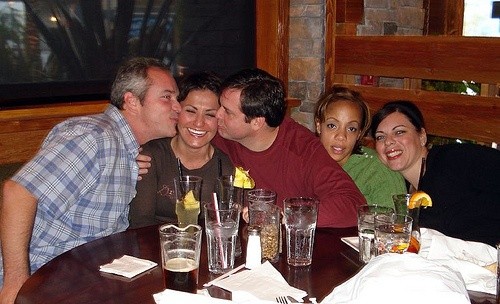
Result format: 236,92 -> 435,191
14,222 -> 500,303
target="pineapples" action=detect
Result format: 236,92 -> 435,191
233,166 -> 256,188
183,190 -> 200,209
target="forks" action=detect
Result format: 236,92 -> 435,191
275,296 -> 288,304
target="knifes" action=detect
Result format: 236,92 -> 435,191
287,295 -> 300,303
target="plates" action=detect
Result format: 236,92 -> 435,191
341,237 -> 359,252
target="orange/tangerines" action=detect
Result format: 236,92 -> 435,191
409,192 -> 432,209
391,244 -> 409,253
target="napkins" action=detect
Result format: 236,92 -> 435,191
99,255 -> 159,279
213,260 -> 308,303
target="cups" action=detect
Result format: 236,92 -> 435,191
158,222 -> 203,294
496,245 -> 500,304
173,175 -> 202,232
247,189 -> 277,221
203,201 -> 242,275
356,204 -> 395,265
215,174 -> 244,258
282,197 -> 320,268
248,201 -> 282,265
374,213 -> 413,257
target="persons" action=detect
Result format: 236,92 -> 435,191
0,56 -> 182,304
313,86 -> 407,223
370,100 -> 500,249
136,68 -> 369,228
128,69 -> 237,230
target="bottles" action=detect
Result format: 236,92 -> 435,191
391,193 -> 422,254
245,224 -> 263,270
